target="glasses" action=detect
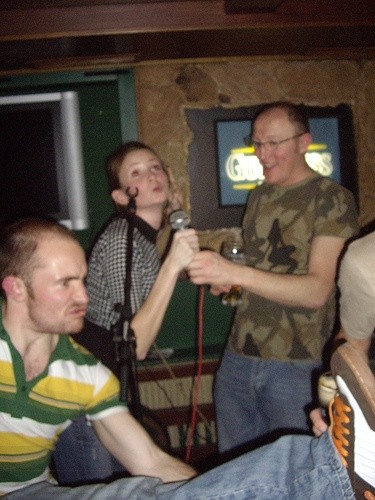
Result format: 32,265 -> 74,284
251,134 -> 303,152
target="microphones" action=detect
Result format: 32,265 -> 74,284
169,210 -> 191,231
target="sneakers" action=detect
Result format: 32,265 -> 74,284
328,341 -> 375,500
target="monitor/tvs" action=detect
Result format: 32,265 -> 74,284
214,117 -> 345,209
0,92 -> 89,232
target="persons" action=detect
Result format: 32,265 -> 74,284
310,230 -> 375,437
52,141 -> 200,488
0,216 -> 375,500
185,102 -> 361,463
